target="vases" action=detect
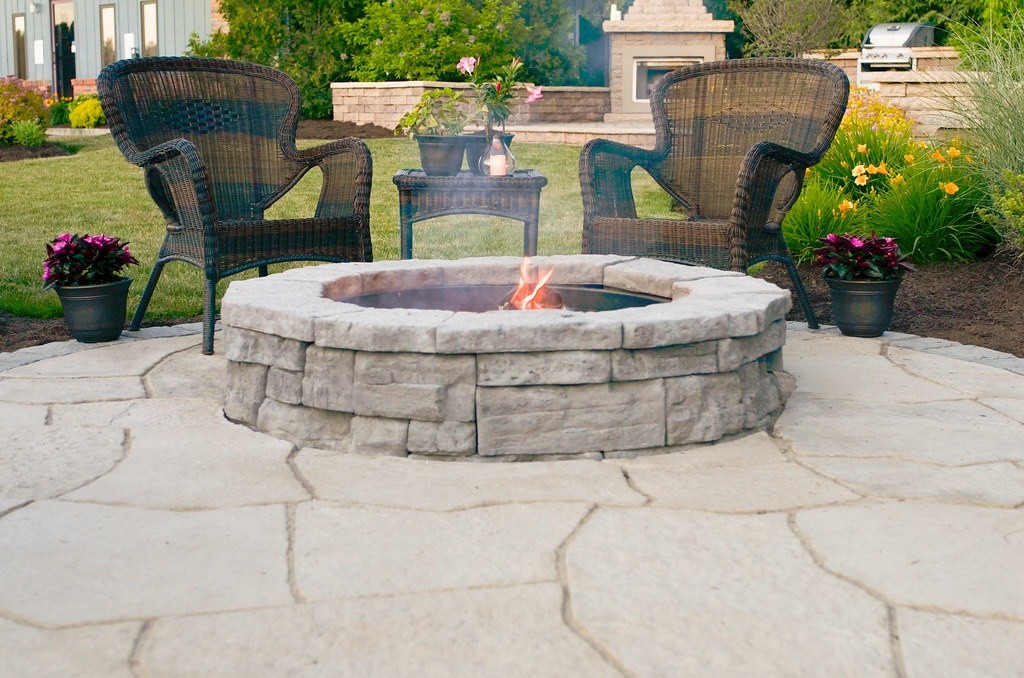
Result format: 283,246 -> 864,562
52,275 -> 134,343
461,132 -> 516,175
824,276 -> 903,337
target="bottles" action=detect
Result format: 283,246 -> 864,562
477,115 -> 516,177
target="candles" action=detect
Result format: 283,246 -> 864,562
490,154 -> 507,176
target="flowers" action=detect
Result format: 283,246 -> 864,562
812,230 -> 919,285
456,55 -> 545,135
41,231 -> 141,292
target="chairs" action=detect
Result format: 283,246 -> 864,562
95,55 -> 375,357
578,55 -> 850,330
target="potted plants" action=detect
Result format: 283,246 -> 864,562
392,86 -> 475,178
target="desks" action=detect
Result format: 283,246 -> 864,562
392,168 -> 548,259
